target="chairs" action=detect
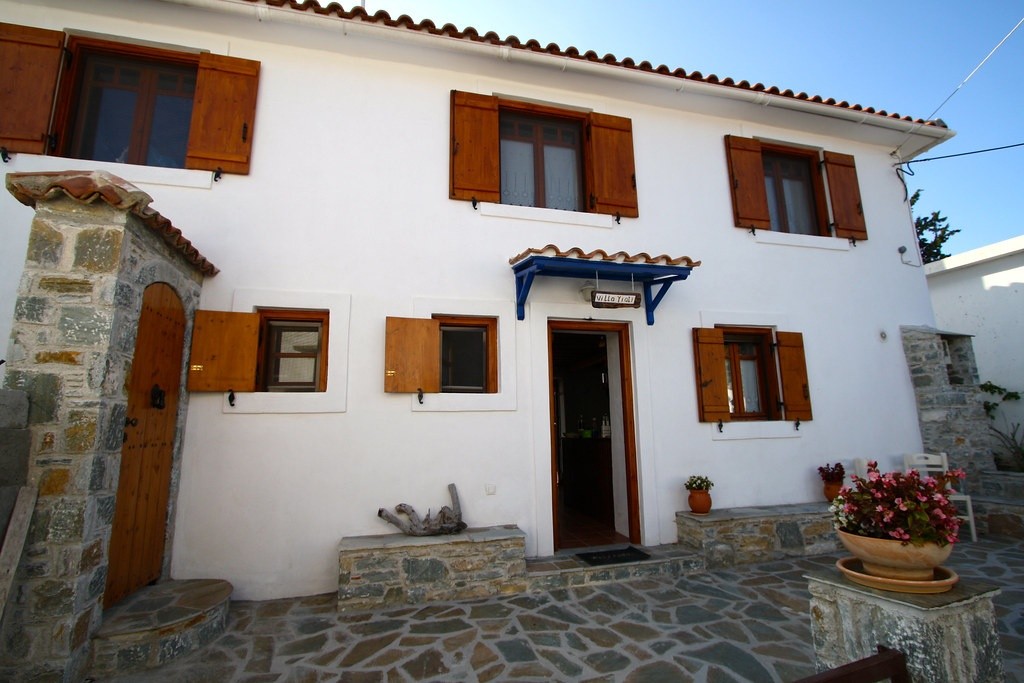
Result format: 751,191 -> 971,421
903,453 -> 977,543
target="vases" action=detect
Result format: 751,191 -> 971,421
837,527 -> 954,580
688,489 -> 712,513
823,481 -> 843,502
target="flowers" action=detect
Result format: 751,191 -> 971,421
684,475 -> 714,490
817,463 -> 845,483
828,458 -> 967,549
